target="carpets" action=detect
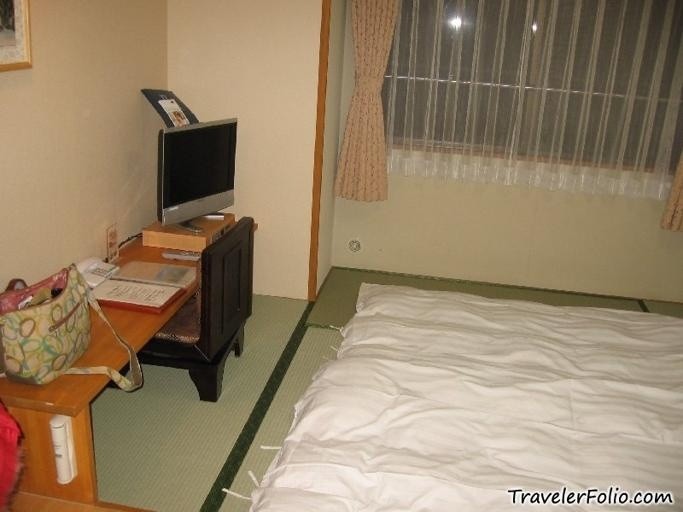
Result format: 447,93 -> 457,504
304,265 -> 683,327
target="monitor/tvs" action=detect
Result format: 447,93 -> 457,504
155,116 -> 239,233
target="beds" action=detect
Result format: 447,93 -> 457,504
222,283 -> 683,512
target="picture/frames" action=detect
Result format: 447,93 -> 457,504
0,0 -> 33,72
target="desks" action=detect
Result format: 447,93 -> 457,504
0,215 -> 256,512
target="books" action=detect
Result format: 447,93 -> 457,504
88,260 -> 198,316
161,248 -> 201,262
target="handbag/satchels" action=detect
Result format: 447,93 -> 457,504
0,263 -> 144,392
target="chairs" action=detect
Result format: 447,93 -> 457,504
110,217 -> 255,402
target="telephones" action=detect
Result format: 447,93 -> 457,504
77,256 -> 120,288
